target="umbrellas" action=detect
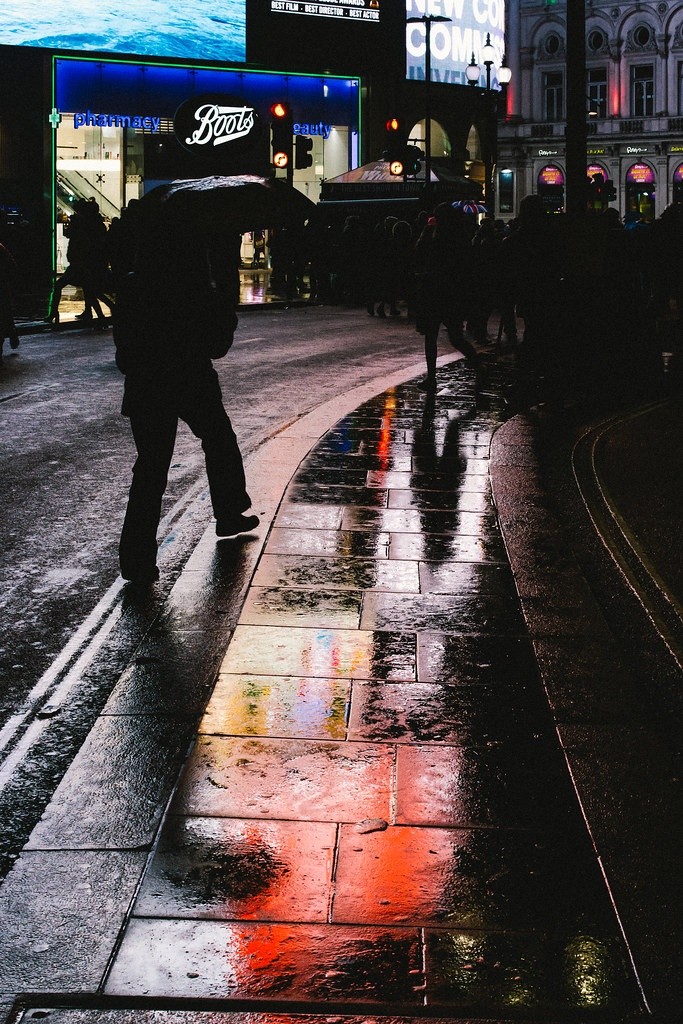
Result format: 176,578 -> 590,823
108,174 -> 317,229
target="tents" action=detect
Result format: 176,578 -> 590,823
318,153 -> 484,206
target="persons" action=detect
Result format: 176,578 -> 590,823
512,194 -> 682,378
362,202 -> 524,391
43,198 -> 114,331
109,226 -> 259,583
252,231 -> 265,264
264,213 -> 371,308
0,243 -> 20,357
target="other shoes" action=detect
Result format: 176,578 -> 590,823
123,566 -> 159,579
377,305 -> 386,318
390,308 -> 400,315
216,515 -> 259,536
366,301 -> 375,315
417,378 -> 436,390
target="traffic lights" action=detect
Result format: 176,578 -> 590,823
296,135 -> 313,170
385,115 -> 423,176
271,102 -> 293,168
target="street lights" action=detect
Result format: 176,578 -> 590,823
466,32 -> 512,220
406,14 -> 454,209
